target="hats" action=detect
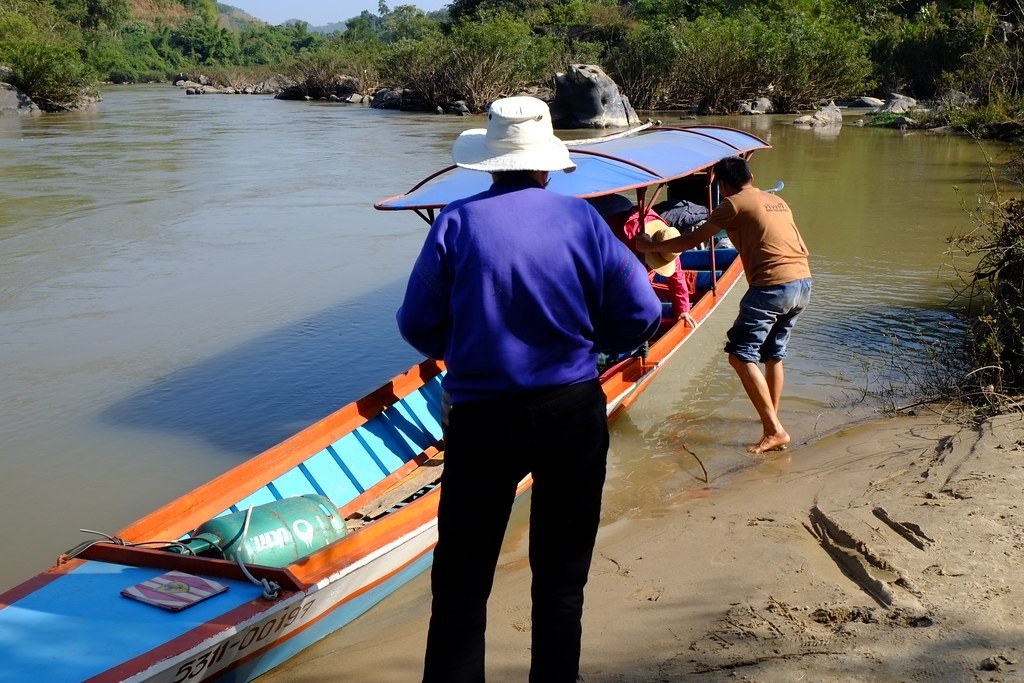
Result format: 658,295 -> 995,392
450,94 -> 580,175
641,219 -> 685,278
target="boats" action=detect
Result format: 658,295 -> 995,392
0,125 -> 786,683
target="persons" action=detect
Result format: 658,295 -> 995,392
395,96 -> 663,683
609,206 -> 698,328
636,157 -> 812,454
